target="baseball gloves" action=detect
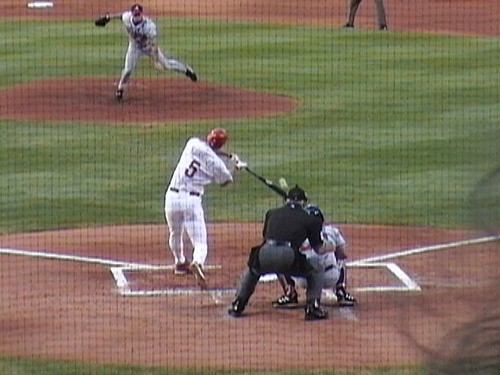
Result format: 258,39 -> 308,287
96,12 -> 110,26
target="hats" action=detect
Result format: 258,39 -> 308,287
287,184 -> 307,201
131,5 -> 142,15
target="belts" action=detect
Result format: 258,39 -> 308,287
170,188 -> 199,196
325,265 -> 333,271
266,239 -> 291,247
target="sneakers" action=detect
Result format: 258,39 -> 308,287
305,309 -> 329,320
272,293 -> 298,307
186,64 -> 197,82
189,263 -> 207,289
228,306 -> 241,317
175,256 -> 191,274
338,293 -> 357,305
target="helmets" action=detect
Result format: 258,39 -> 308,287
305,205 -> 324,223
208,128 -> 227,148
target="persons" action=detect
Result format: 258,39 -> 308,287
345,0 -> 389,31
164,128 -> 247,289
95,4 -> 197,102
229,184 -> 329,320
272,203 -> 358,308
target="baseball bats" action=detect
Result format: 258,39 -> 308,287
230,155 -> 286,199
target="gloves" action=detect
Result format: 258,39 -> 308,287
229,153 -> 247,171
